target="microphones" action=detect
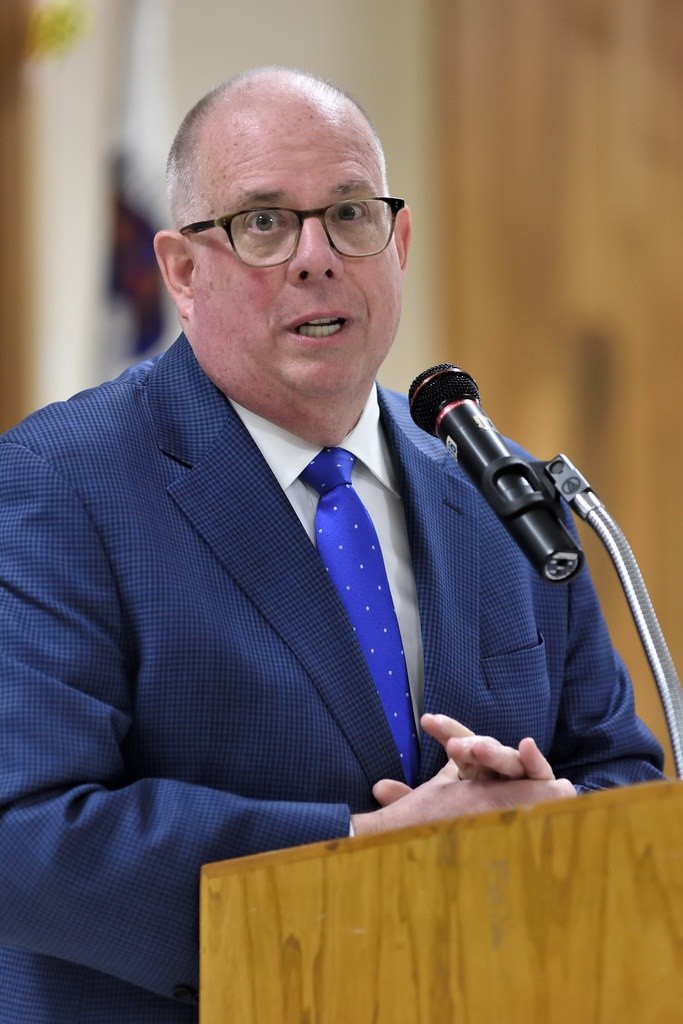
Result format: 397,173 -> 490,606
409,363 -> 584,581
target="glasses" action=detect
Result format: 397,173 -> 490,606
177,196 -> 403,266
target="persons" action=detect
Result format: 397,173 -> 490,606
1,66 -> 666,1023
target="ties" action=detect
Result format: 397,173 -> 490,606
297,444 -> 420,785
